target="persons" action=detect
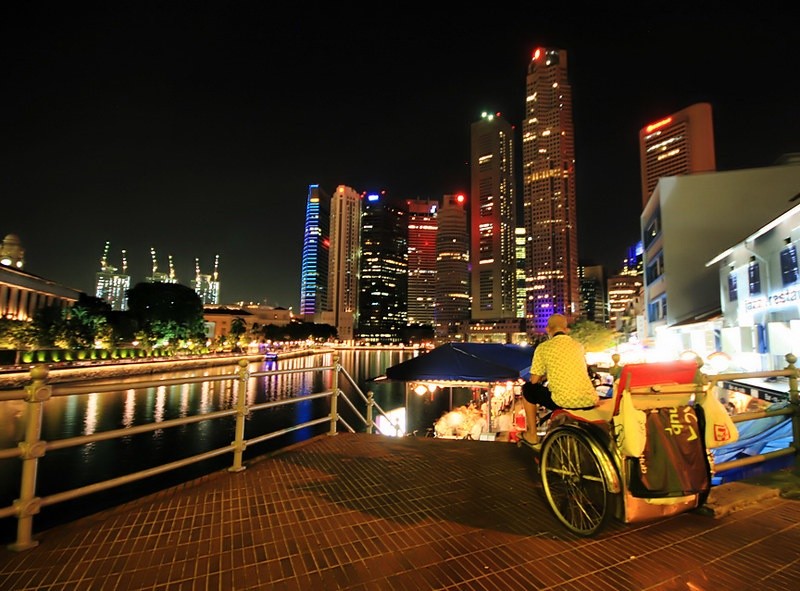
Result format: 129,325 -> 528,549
515,313 -> 600,445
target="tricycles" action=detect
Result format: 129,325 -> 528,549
514,362 -> 711,537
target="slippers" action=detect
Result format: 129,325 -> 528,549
515,430 -> 538,446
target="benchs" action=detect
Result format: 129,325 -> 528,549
559,360 -> 701,435
